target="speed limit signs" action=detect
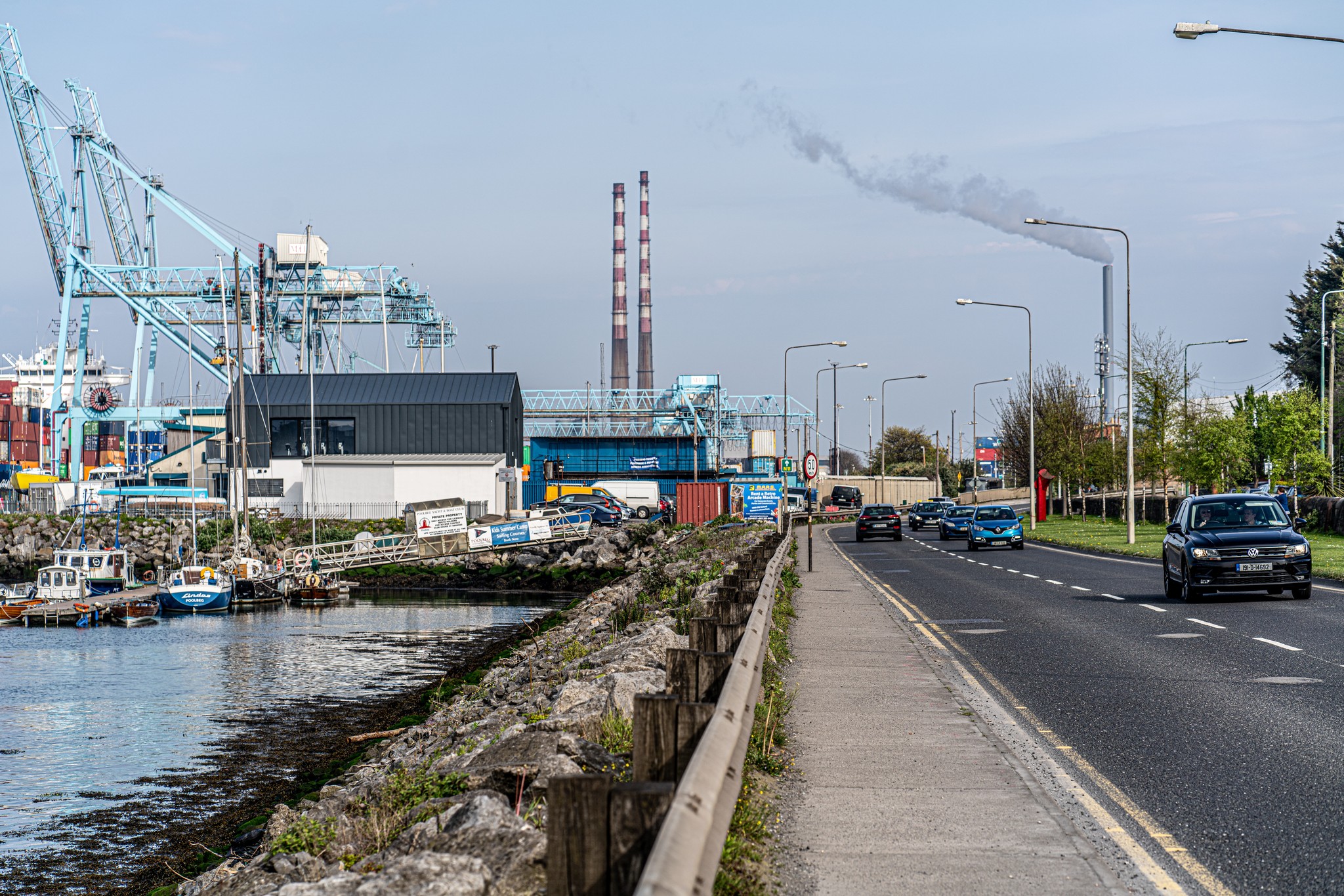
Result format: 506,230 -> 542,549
805,452 -> 818,479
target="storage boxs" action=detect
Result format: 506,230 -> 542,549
0,380 -> 167,485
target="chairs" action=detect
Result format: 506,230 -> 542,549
883,509 -> 889,514
866,509 -> 873,515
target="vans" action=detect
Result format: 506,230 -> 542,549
782,486 -> 819,512
829,485 -> 864,510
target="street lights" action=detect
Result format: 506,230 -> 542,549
932,430 -> 939,497
863,395 -> 877,476
1038,369 -> 1231,508
784,341 -> 848,510
973,377 -> 1013,505
960,422 -> 978,461
1184,338 -> 1249,495
1024,217 -> 1137,545
955,298 -> 1039,531
951,410 -> 956,464
488,345 -> 499,373
791,428 -> 802,483
882,373 -> 927,503
815,362 -> 868,510
832,404 -> 844,475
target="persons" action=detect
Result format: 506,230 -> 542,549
1001,512 -> 1007,520
883,508 -> 889,513
1242,505 -> 1267,525
950,511 -> 958,518
969,511 -> 980,517
1274,487 -> 1290,520
932,505 -> 937,512
918,505 -> 927,512
1195,506 -> 1216,528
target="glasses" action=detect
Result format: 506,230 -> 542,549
1202,510 -> 1211,514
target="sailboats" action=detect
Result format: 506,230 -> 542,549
0,225 -> 352,628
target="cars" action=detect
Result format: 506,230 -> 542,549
908,496 -> 1024,552
1231,480 -> 1308,500
1073,483 -> 1099,495
528,479 -> 678,535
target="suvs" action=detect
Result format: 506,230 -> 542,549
855,503 -> 902,542
1162,491 -> 1312,603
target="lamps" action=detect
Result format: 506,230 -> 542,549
81,544 -> 86,550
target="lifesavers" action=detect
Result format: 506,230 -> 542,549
295,552 -> 311,568
73,603 -> 90,612
143,570 -> 154,581
89,501 -> 99,512
103,548 -> 116,550
200,567 -> 214,579
306,574 -> 319,587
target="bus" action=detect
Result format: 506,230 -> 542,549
962,477 -> 1003,493
